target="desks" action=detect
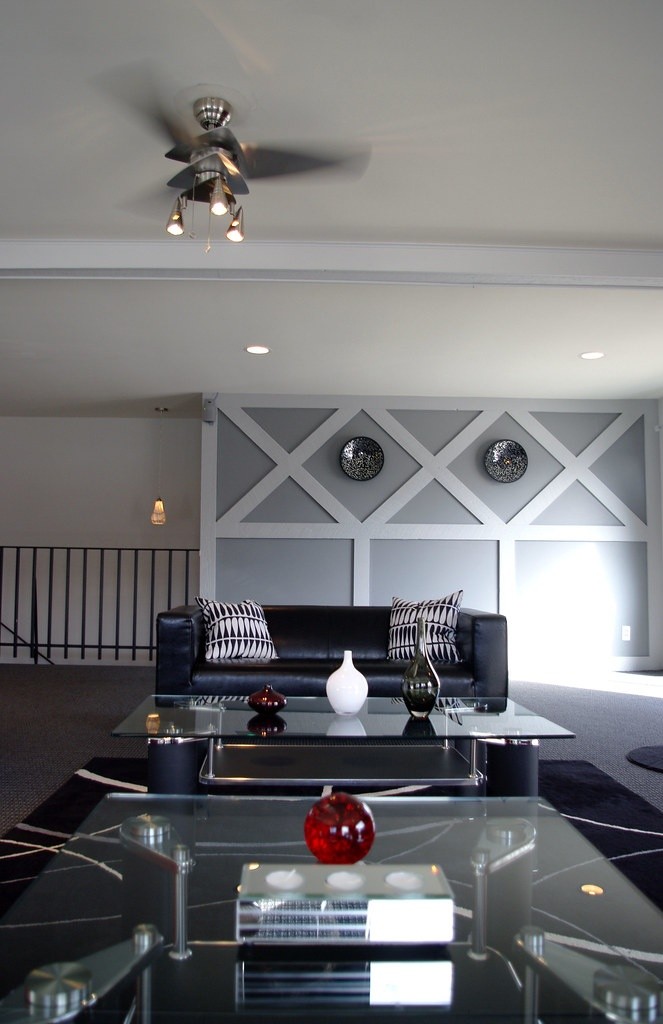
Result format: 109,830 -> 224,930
0,793 -> 663,1024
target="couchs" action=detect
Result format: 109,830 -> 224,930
156,605 -> 509,698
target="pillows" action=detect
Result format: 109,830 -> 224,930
193,595 -> 280,659
386,590 -> 464,663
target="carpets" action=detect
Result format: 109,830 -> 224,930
0,757 -> 663,1024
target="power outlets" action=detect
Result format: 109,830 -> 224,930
621,626 -> 631,640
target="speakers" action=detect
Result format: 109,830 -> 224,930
203,399 -> 216,422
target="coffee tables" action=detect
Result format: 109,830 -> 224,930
111,695 -> 575,798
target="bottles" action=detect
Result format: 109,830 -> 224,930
326,651 -> 368,714
401,617 -> 440,719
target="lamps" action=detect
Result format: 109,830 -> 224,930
150,408 -> 168,525
166,171 -> 244,254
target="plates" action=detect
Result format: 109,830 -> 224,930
339,436 -> 384,481
484,439 -> 528,483
